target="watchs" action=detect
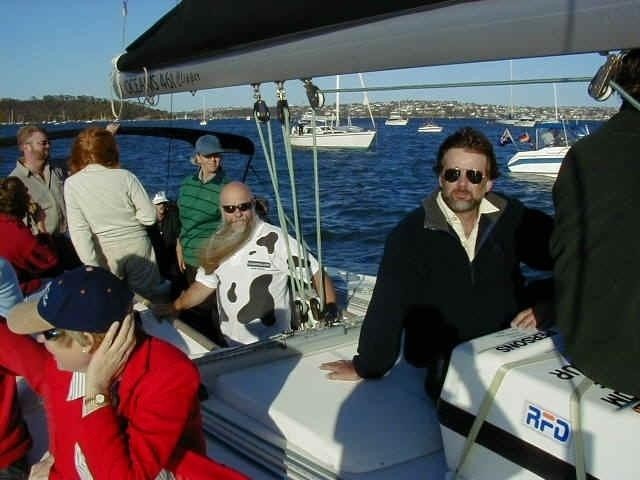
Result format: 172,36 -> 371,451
83,392 -> 113,407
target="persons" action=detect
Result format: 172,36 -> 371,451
540,127 -> 556,147
545,46 -> 640,402
5,120 -> 121,240
172,133 -> 236,335
0,265 -> 252,479
144,189 -> 178,264
60,125 -> 170,304
315,124 -> 557,408
151,178 -> 342,349
0,175 -> 61,297
0,256 -> 26,478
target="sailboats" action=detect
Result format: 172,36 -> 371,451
199,94 -> 207,126
495,60 -> 536,128
289,73 -> 377,150
537,82 -> 562,125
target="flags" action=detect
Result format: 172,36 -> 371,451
518,131 -> 531,144
495,127 -> 514,147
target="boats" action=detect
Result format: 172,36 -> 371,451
508,123 -> 592,178
0,2 -> 640,479
385,103 -> 409,126
416,122 -> 444,134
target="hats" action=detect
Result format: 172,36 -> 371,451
152,191 -> 170,204
195,135 -> 224,155
7,266 -> 134,335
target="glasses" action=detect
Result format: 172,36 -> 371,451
43,329 -> 65,341
442,169 -> 483,184
222,202 -> 251,213
